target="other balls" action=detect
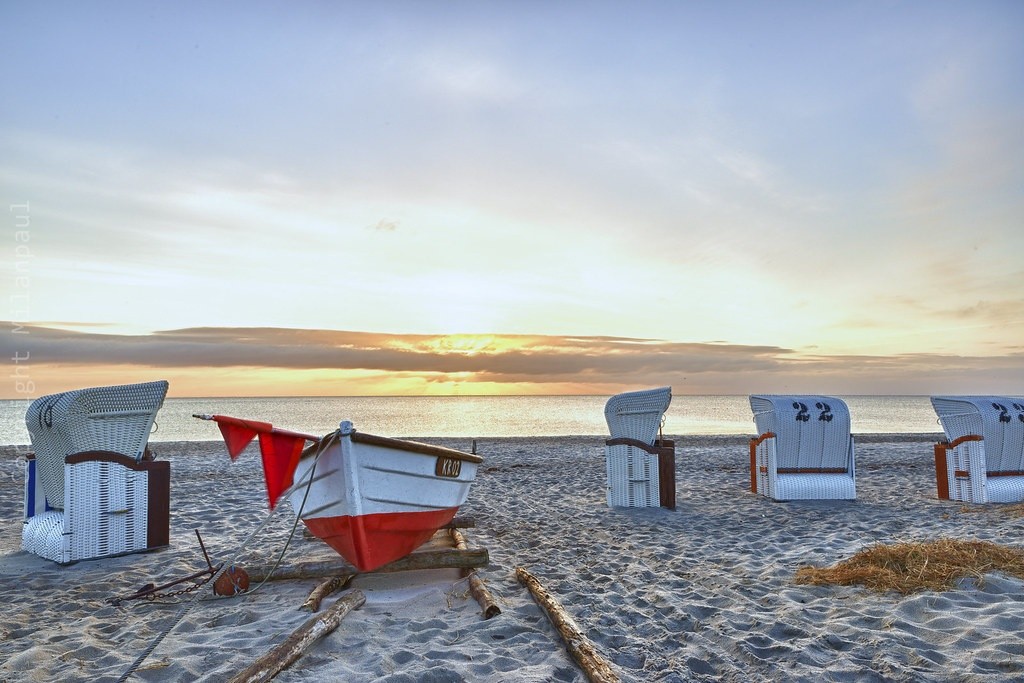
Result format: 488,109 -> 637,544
214,565 -> 250,596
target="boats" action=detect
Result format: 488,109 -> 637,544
286,419 -> 484,573
